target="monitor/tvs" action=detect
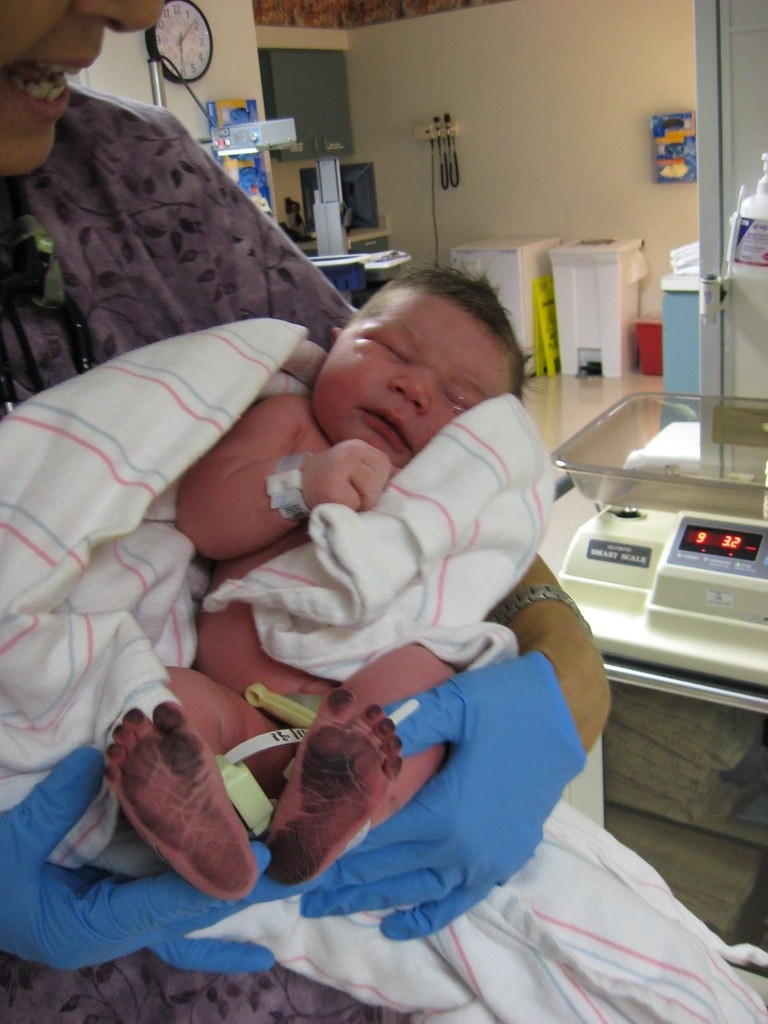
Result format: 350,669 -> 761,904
297,162 -> 378,232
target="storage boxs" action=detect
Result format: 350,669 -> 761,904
635,313 -> 663,375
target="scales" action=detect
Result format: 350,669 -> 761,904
547,392 -> 768,688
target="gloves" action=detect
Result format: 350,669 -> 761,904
300,650 -> 587,941
0,746 -> 323,975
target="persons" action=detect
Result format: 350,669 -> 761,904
0,0 -> 608,1021
92,268 -> 533,899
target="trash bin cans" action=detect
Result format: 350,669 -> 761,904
634,322 -> 663,376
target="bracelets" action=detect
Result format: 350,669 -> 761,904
492,584 -> 592,638
266,453 -> 310,520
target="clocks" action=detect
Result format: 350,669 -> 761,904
145,0 -> 212,84
